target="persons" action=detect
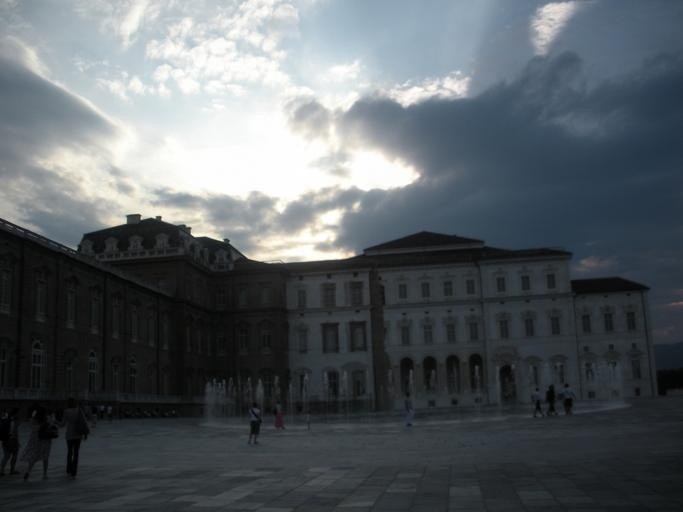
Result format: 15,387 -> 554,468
403,392 -> 414,427
558,384 -> 576,416
305,410 -> 312,433
273,399 -> 285,431
247,402 -> 262,444
0,409 -> 19,476
19,400 -> 57,481
546,384 -> 558,416
530,387 -> 545,418
53,396 -> 90,480
85,402 -> 113,429
